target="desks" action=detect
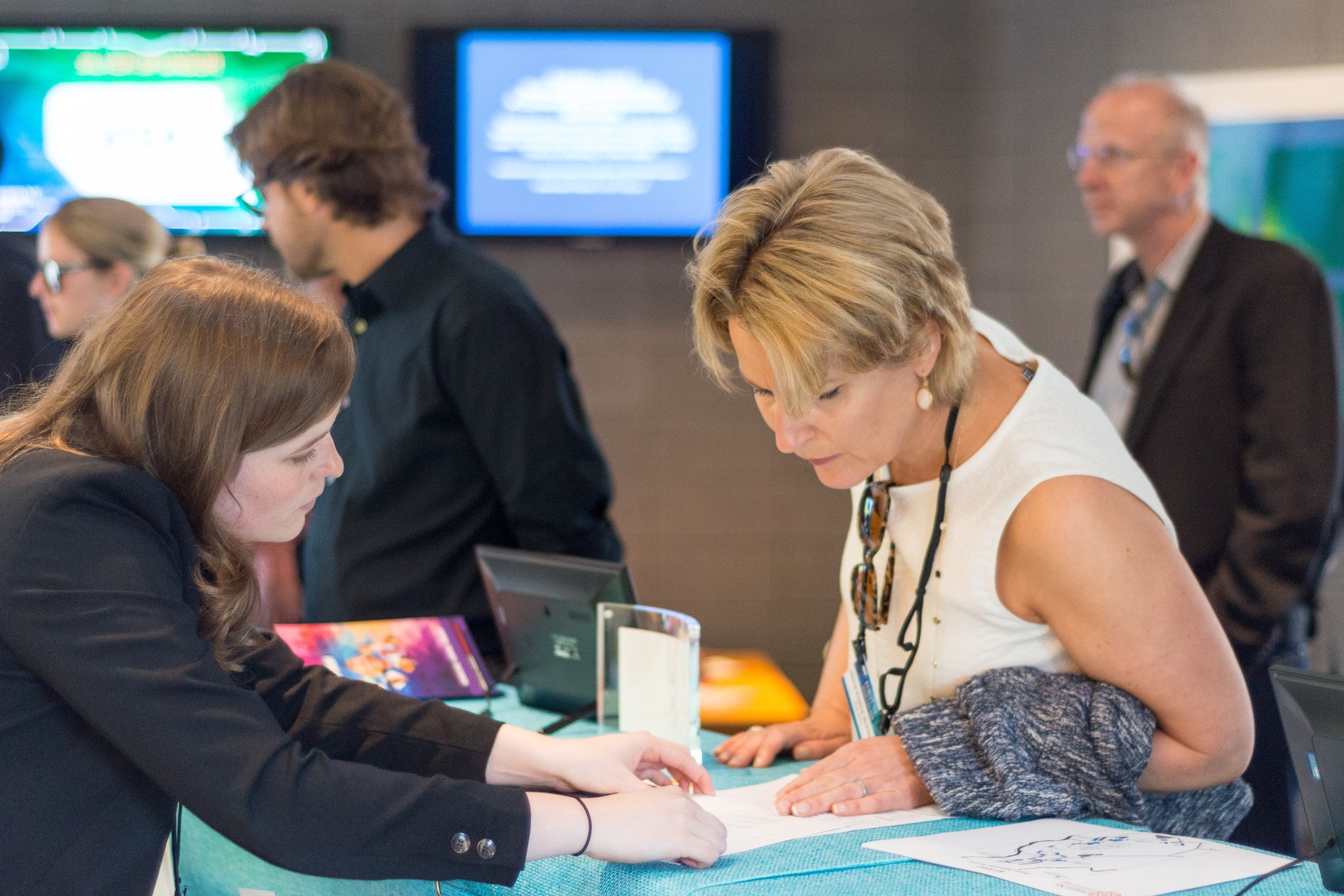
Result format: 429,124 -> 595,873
150,683 -> 1344,896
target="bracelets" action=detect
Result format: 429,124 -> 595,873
569,794 -> 592,857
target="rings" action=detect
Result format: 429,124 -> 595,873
750,727 -> 764,732
854,780 -> 868,795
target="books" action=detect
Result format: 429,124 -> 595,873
273,615 -> 503,702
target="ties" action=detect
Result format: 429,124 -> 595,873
1117,279 -> 1167,388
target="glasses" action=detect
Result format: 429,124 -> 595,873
41,261 -> 112,294
851,480 -> 896,630
1067,141 -> 1187,172
237,156 -> 322,216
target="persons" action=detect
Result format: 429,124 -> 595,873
224,59 -> 636,685
684,146 -> 1254,839
1065,70 -> 1344,858
30,197 -> 308,624
0,256 -> 728,896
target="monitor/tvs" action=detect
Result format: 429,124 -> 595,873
0,22 -> 339,240
473,541 -> 639,722
1268,664 -> 1344,894
441,21 -> 773,242
